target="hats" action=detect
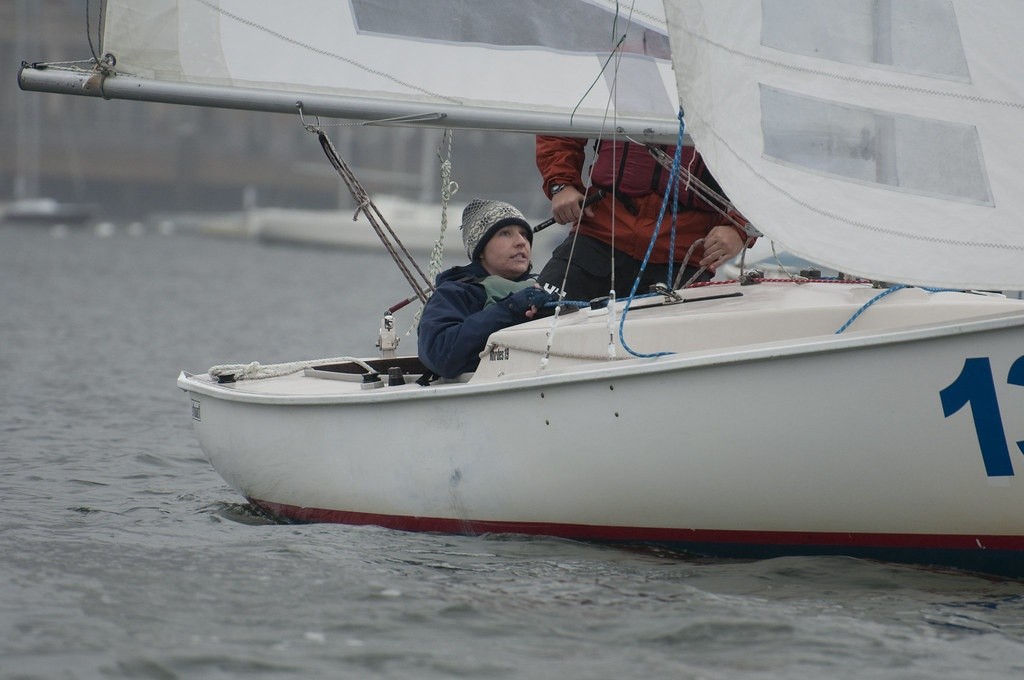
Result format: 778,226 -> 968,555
462,198 -> 534,263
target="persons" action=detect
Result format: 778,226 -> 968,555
532,132 -> 760,321
414,197 -> 583,378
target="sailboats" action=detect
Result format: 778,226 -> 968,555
14,1 -> 1024,571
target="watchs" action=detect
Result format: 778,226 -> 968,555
549,182 -> 566,195
